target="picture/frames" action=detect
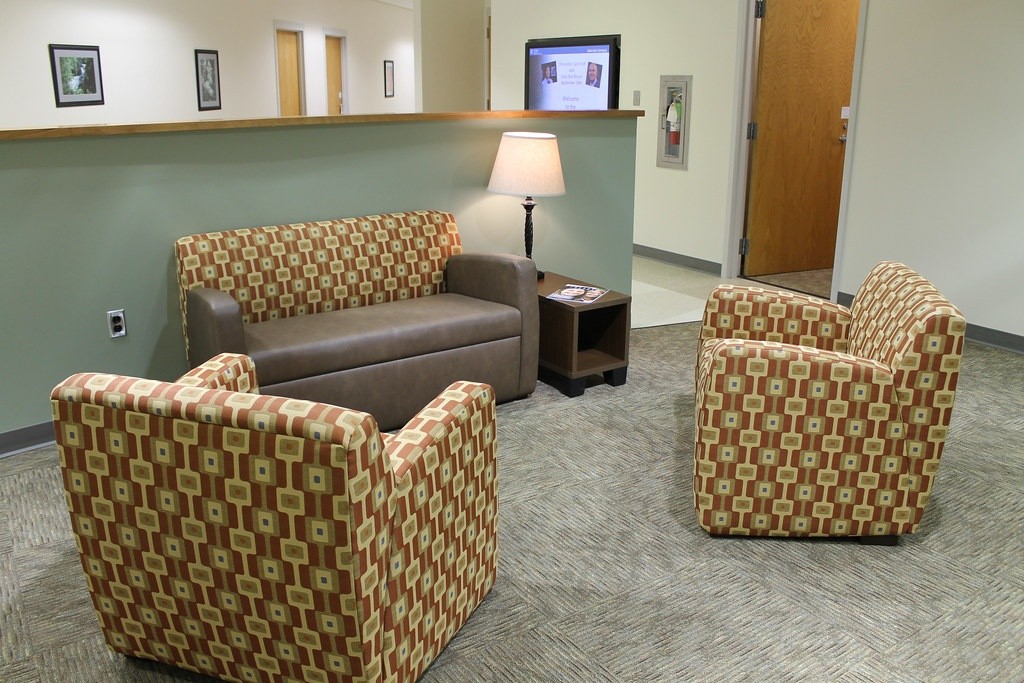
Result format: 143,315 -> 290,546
384,60 -> 395,97
49,44 -> 104,107
195,49 -> 222,111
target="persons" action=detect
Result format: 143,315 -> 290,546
542,67 -> 553,84
77,62 -> 89,94
587,64 -> 599,88
550,286 -> 601,301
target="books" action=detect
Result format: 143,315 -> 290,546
546,283 -> 610,303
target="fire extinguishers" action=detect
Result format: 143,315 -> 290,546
668,93 -> 683,145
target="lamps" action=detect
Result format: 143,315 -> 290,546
486,131 -> 565,280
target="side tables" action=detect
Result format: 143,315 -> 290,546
535,272 -> 632,397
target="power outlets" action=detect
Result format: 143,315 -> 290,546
106,309 -> 126,338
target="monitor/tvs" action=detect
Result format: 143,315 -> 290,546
524,33 -> 622,111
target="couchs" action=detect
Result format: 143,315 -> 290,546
175,210 -> 541,435
693,260 -> 968,545
50,351 -> 501,683
